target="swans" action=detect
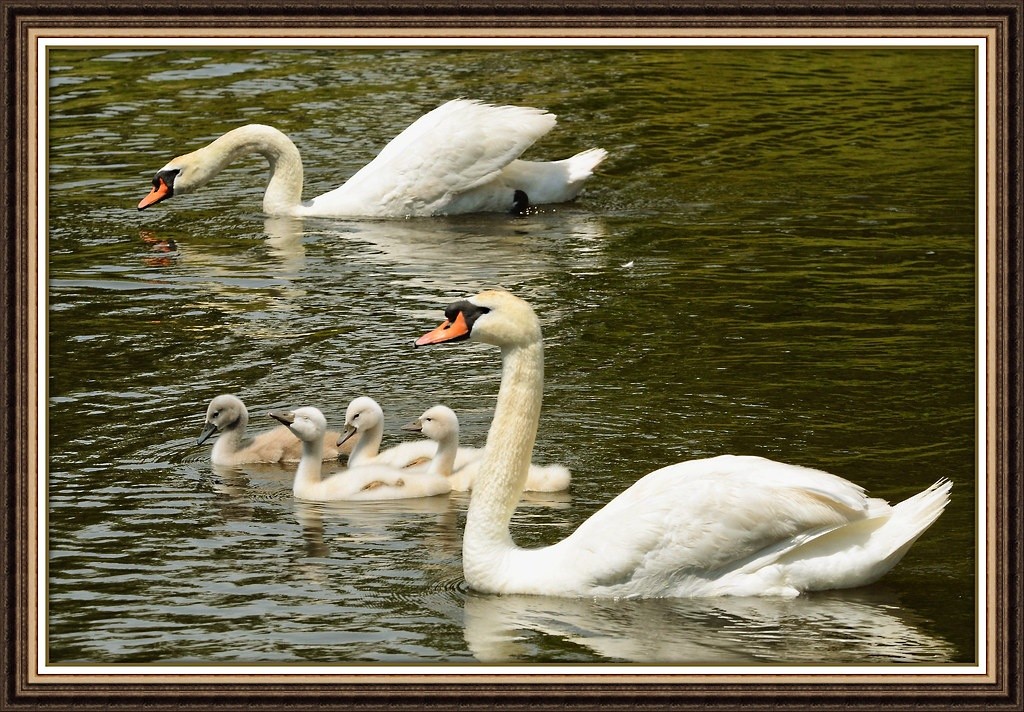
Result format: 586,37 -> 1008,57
137,97 -> 609,217
413,289 -> 955,598
197,394 -> 570,503
